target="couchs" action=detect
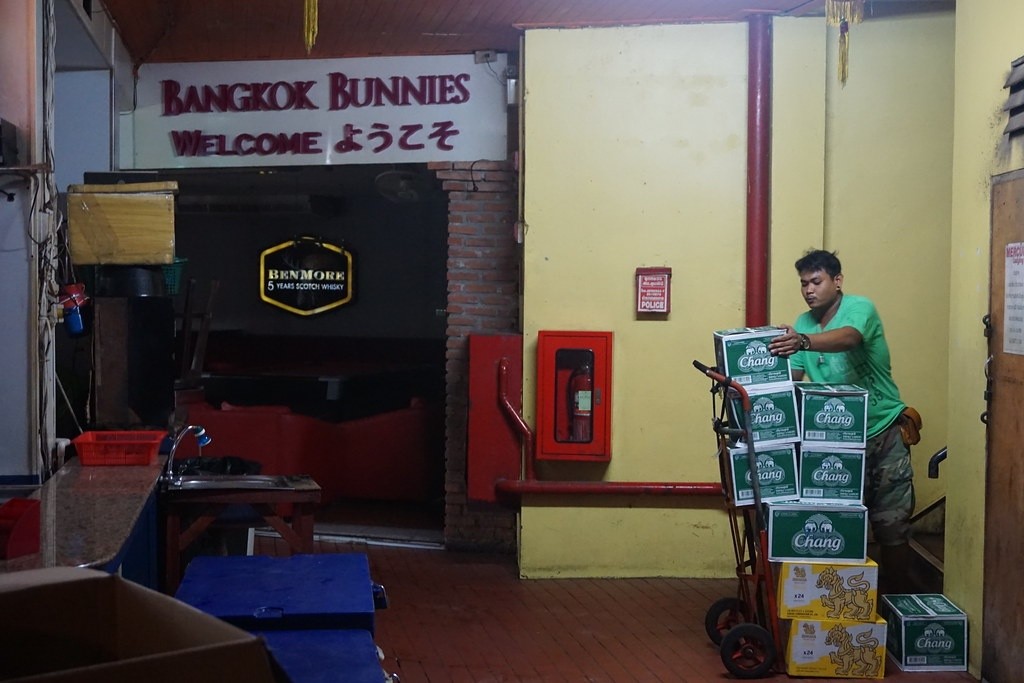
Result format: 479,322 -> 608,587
174,388 -> 427,501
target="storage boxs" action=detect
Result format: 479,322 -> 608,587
71,432 -> 169,465
66,181 -> 178,266
0,567 -> 272,683
713,326 -> 968,679
175,553 -> 386,683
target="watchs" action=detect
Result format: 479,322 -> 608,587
798,333 -> 811,351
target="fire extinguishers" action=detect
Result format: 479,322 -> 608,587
568,354 -> 592,442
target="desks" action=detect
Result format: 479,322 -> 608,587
165,476 -> 321,595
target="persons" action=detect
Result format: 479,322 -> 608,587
766,249 -> 919,594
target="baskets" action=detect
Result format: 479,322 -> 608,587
70,430 -> 169,466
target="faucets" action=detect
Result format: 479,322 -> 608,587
165,424 -> 211,475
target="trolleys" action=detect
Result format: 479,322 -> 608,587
691,358 -> 786,681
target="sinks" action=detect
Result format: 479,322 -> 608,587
167,471 -> 294,492
